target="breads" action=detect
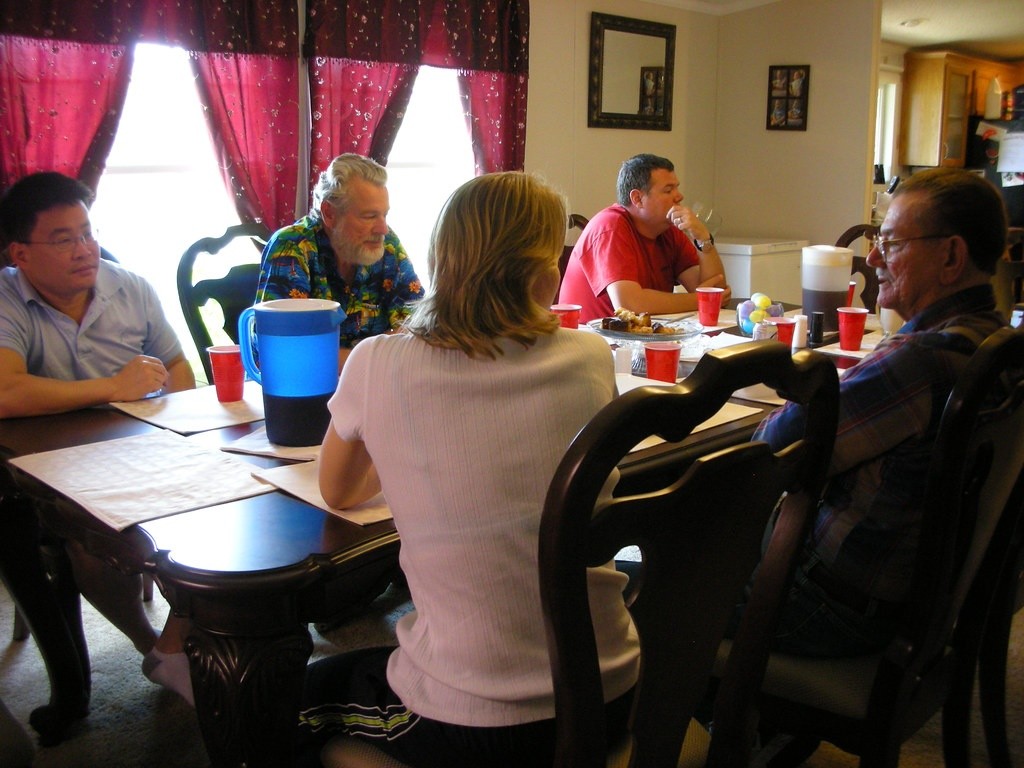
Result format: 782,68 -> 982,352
601,307 -> 675,334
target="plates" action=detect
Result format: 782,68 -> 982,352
586,316 -> 705,341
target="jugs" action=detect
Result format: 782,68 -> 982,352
801,244 -> 854,332
238,298 -> 348,448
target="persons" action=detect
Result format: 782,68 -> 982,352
559,154 -> 731,323
649,168 -> 1024,767
254,154 -> 423,376
645,72 -> 664,114
280,172 -> 640,768
0,171 -> 196,710
770,69 -> 805,126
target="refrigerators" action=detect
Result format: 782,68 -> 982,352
674,237 -> 810,337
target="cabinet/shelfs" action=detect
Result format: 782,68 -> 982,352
896,50 -> 1024,168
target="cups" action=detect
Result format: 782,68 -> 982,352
809,310 -> 825,344
791,314 -> 807,349
847,281 -> 857,307
550,304 -> 582,330
763,316 -> 797,348
880,308 -> 904,336
643,342 -> 682,383
206,345 -> 245,403
836,306 -> 870,351
695,287 -> 724,326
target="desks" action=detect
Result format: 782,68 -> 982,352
0,296 -> 884,768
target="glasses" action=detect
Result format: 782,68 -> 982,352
20,230 -> 98,250
872,232 -> 963,256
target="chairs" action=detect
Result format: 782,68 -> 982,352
308,338 -> 840,768
696,326 -> 1024,768
177,223 -> 274,385
553,215 -> 589,306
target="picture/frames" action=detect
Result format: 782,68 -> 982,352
766,65 -> 810,131
637,66 -> 665,116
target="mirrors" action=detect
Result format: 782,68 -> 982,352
587,11 -> 678,131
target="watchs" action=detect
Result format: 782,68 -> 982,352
694,234 -> 714,251
